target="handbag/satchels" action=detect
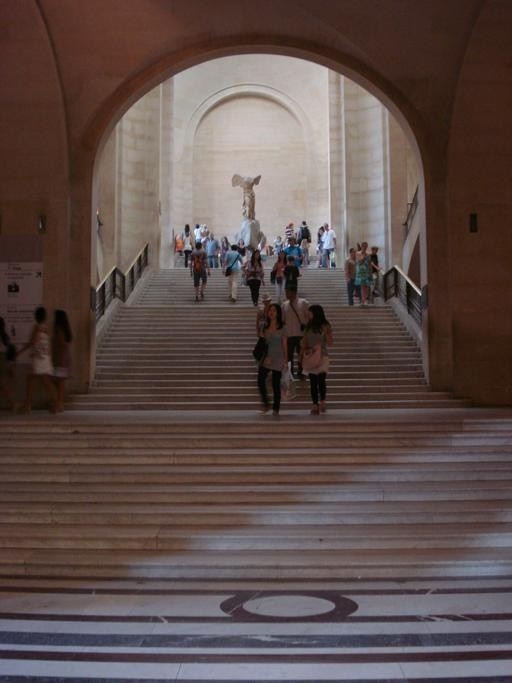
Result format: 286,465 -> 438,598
224,267 -> 232,276
253,337 -> 268,364
303,344 -> 322,369
301,323 -> 305,331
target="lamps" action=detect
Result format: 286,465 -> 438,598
26,181 -> 52,235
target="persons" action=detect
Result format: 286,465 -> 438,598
241,177 -> 257,218
0,319 -> 21,410
173,219 -> 383,375
255,306 -> 290,415
298,302 -> 334,417
16,307 -> 52,415
50,309 -> 75,407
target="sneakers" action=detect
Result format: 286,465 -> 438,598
320,402 -> 326,413
311,404 -> 319,416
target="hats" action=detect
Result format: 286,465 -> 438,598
261,292 -> 271,302
